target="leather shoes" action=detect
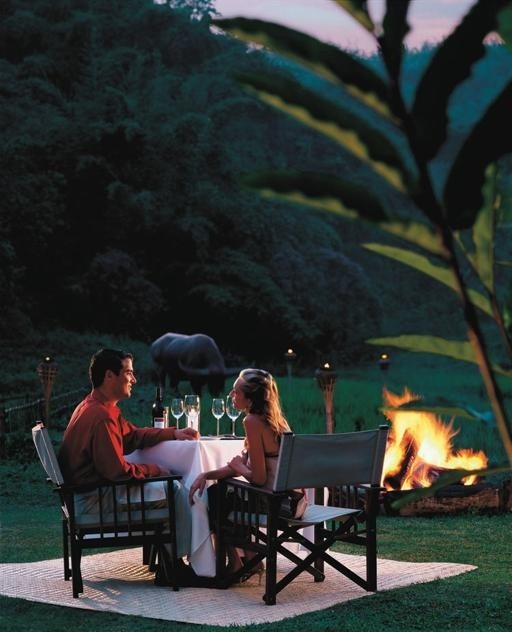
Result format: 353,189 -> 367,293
154,562 -> 203,588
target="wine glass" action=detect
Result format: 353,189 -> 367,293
183,395 -> 201,427
170,398 -> 184,429
211,398 -> 225,436
225,394 -> 242,436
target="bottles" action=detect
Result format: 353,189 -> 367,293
151,386 -> 165,429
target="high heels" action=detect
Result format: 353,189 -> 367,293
242,560 -> 265,584
208,562 -> 246,590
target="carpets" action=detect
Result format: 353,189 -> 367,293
1,545 -> 479,626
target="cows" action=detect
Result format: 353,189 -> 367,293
149,330 -> 244,402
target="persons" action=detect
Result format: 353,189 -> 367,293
187,367 -> 307,589
59,347 -> 209,587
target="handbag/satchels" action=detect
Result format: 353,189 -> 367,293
280,490 -> 307,520
